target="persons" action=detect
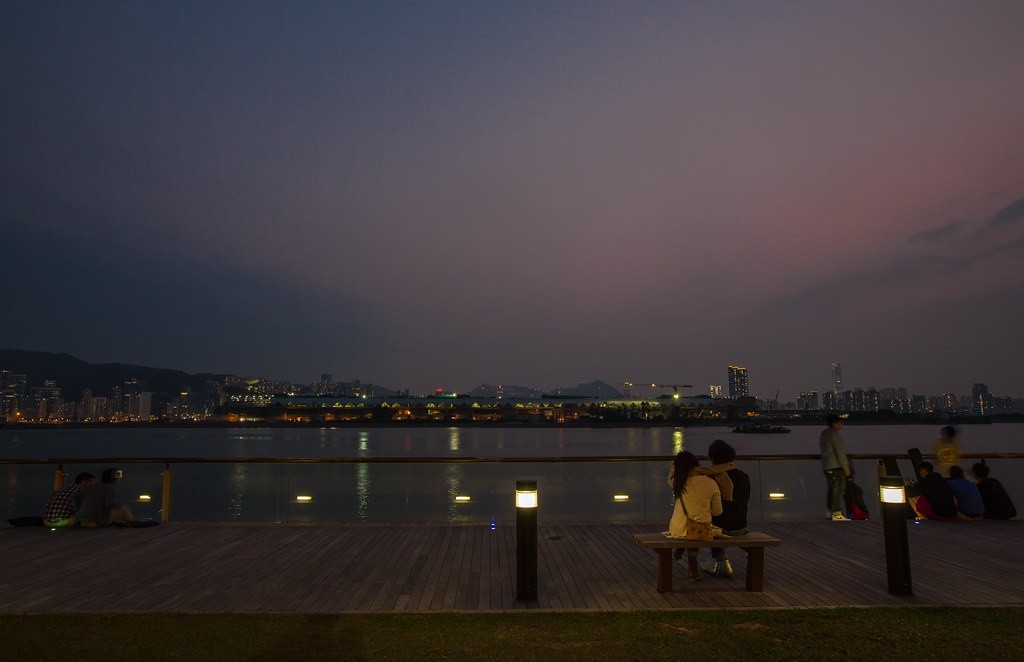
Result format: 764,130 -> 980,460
667,440 -> 751,584
904,425 -> 1017,522
41,468 -> 135,529
820,414 -> 855,522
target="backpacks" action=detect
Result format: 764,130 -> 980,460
844,477 -> 869,520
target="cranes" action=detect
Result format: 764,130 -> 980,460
641,383 -> 692,394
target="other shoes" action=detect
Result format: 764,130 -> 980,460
826,509 -> 852,521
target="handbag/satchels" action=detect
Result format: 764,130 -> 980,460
686,518 -> 713,546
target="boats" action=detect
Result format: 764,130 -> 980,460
732,423 -> 791,433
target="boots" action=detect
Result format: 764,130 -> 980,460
688,556 -> 703,582
702,559 -> 733,577
673,548 -> 684,560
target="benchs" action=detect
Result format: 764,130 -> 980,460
630,531 -> 783,594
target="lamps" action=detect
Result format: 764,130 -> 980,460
515,480 -> 539,508
879,475 -> 905,504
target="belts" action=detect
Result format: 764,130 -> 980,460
45,517 -> 68,524
